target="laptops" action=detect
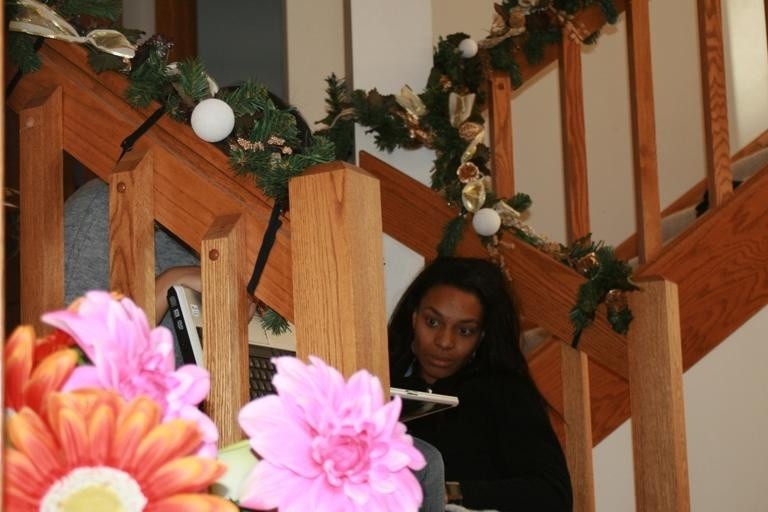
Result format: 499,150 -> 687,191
166,285 -> 460,423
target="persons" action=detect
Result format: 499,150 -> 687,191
382,250 -> 578,511
59,77 -> 316,371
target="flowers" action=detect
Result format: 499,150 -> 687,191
2,290 -> 427,512
